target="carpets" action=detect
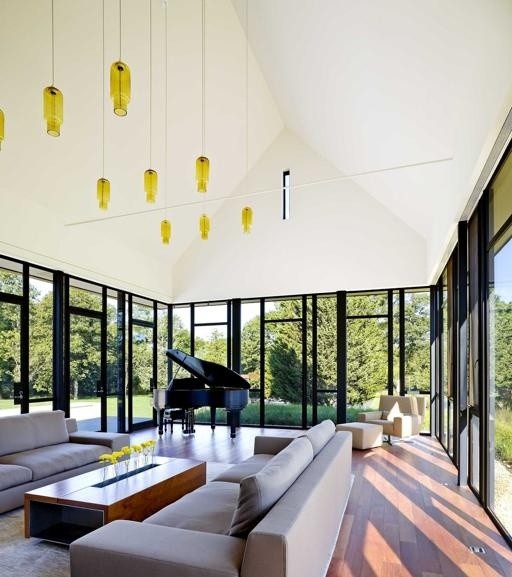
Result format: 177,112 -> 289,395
0,461 -> 235,577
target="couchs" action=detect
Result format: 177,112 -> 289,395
0,409 -> 130,507
66,418 -> 355,577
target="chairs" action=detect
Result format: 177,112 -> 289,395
357,394 -> 425,446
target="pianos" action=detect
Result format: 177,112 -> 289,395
152,348 -> 251,437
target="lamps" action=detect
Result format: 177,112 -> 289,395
161,219 -> 171,243
242,206 -> 252,232
0,107 -> 4,150
195,0 -> 209,193
97,0 -> 110,209
111,0 -> 131,116
200,213 -> 209,239
144,0 -> 157,203
44,0 -> 64,138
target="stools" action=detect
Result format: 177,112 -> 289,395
163,409 -> 185,433
336,421 -> 383,449
